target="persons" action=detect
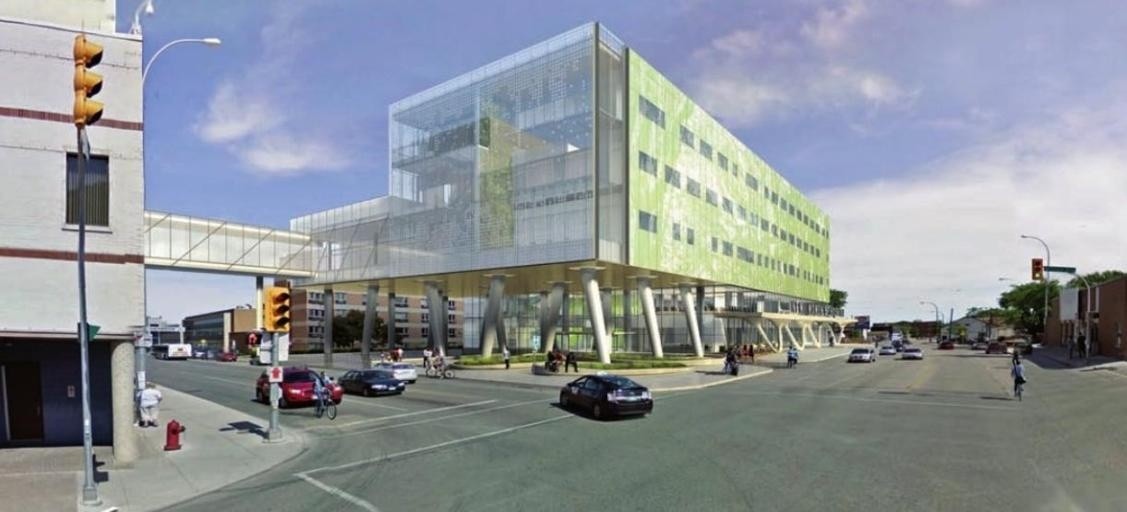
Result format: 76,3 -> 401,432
1068,335 -> 1075,360
545,350 -> 578,372
723,344 -> 754,375
1077,332 -> 1086,359
390,347 -> 449,380
786,346 -> 799,369
1011,361 -> 1024,397
139,383 -> 162,427
503,343 -> 511,369
1012,348 -> 1019,365
314,371 -> 333,410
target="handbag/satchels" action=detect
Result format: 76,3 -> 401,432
1014,374 -> 1026,385
